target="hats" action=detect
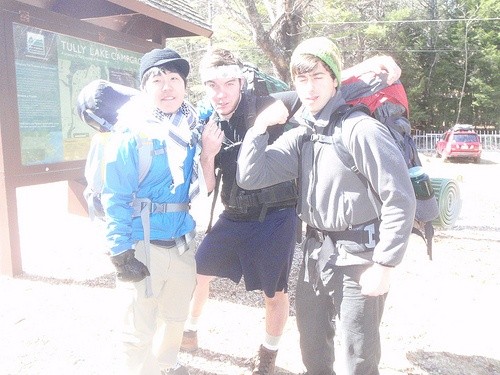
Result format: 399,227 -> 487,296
292,37 -> 342,91
140,48 -> 190,82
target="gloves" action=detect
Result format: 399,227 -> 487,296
112,250 -> 149,282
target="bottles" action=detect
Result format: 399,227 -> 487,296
409,165 -> 434,201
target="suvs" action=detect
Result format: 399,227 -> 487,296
435,128 -> 482,164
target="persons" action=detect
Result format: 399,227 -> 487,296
236,36 -> 417,375
102,47 -> 213,375
180,41 -> 298,375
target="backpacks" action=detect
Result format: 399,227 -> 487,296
301,55 -> 439,222
241,61 -> 293,116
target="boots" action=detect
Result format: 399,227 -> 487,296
180,330 -> 198,351
253,344 -> 278,375
